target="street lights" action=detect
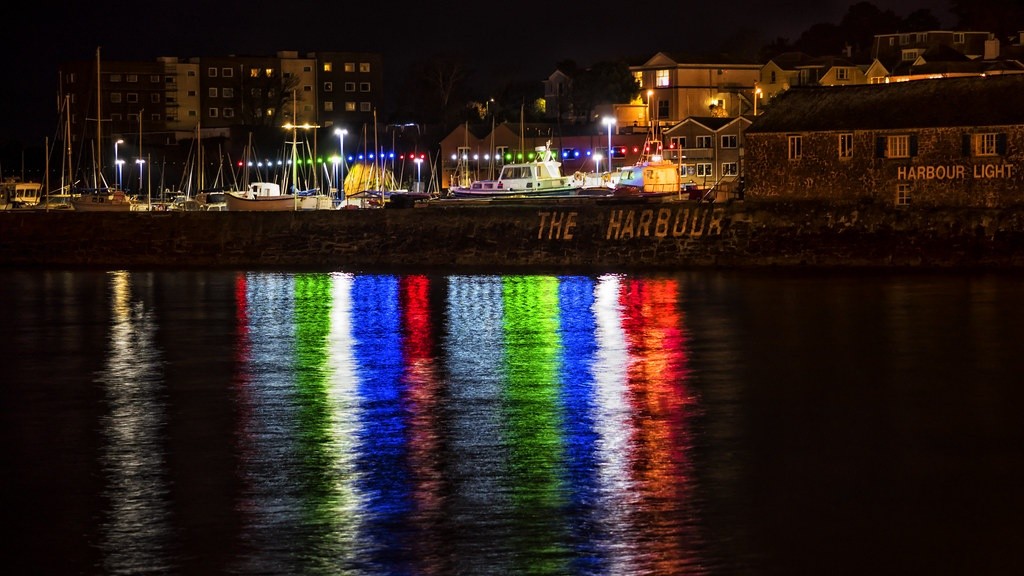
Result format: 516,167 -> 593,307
135,160 -> 147,192
334,128 -> 349,200
114,139 -> 124,190
413,158 -> 424,183
115,160 -> 127,190
602,118 -> 616,172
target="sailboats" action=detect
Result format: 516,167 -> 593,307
0,47 -> 680,210
